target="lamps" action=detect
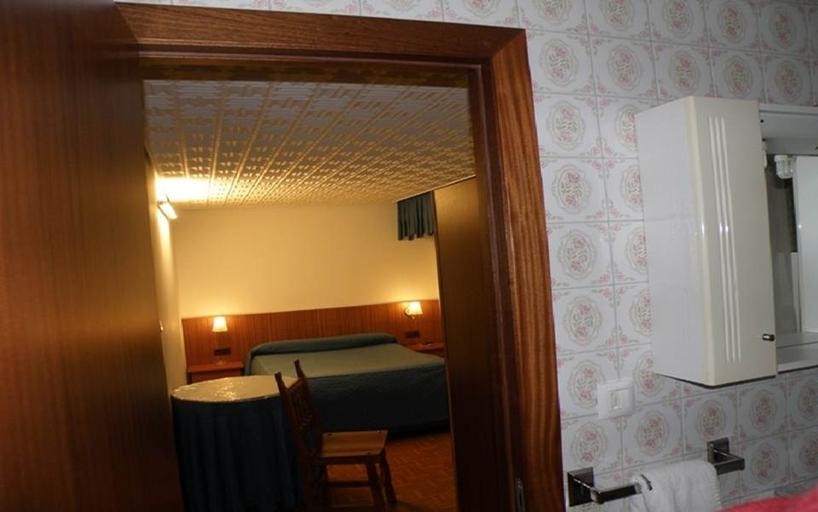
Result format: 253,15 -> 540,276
404,301 -> 424,319
212,317 -> 228,333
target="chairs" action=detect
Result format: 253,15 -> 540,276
275,358 -> 396,512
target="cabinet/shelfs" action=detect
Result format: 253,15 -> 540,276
633,94 -> 779,387
792,154 -> 818,334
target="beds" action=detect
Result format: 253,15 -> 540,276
248,331 -> 449,433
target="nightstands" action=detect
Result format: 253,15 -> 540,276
187,361 -> 244,383
406,342 -> 445,357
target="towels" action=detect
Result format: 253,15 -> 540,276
630,459 -> 722,512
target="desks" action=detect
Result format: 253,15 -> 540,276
171,374 -> 324,512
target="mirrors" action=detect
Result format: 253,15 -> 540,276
766,154 -> 818,347
